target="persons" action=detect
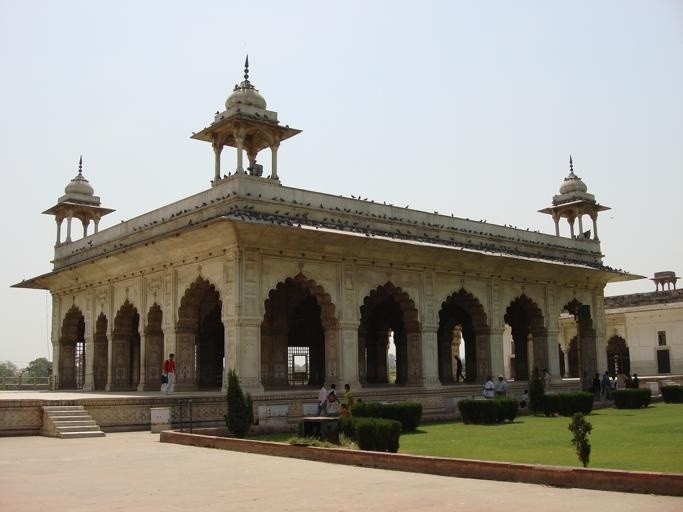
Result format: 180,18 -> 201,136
481,367 -> 641,408
316,381 -> 362,417
455,355 -> 465,382
163,353 -> 177,395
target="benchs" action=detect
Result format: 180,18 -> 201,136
286,416 -> 342,444
